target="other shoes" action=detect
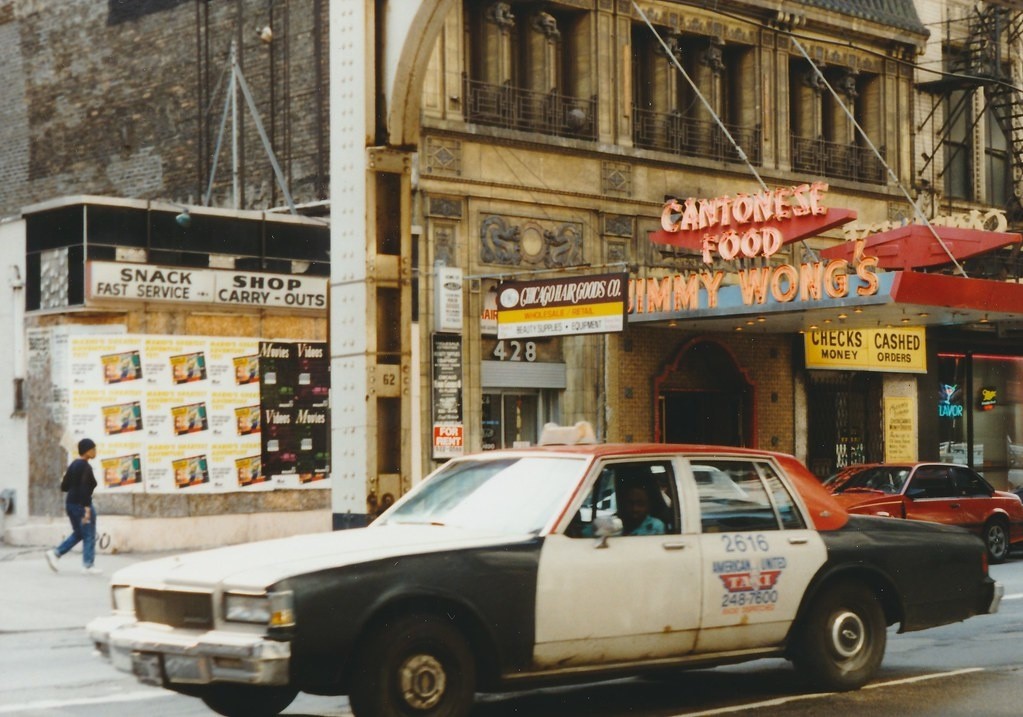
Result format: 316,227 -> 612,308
80,566 -> 102,575
46,550 -> 60,571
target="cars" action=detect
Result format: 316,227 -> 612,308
820,459 -> 1023,564
85,437 -> 1006,717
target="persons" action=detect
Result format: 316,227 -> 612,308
616,484 -> 665,536
45,439 -> 102,573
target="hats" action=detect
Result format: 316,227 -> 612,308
78,439 -> 95,454
